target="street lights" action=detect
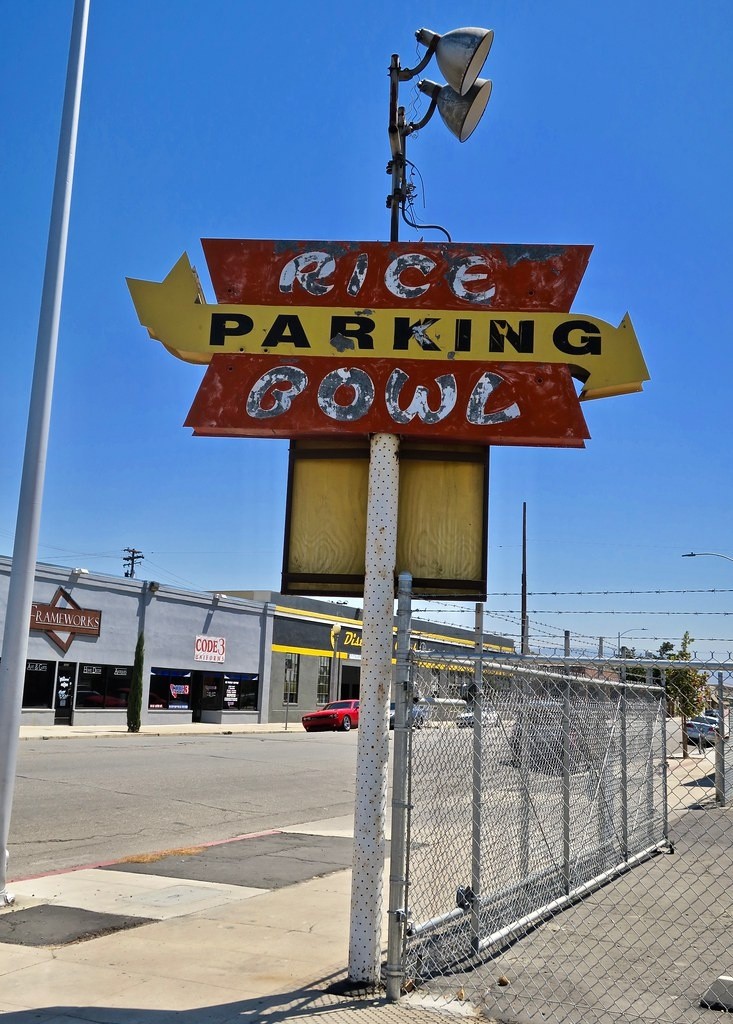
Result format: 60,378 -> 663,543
618,628 -> 649,658
343,17 -> 499,995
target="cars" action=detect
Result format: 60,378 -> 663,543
704,710 -> 719,717
389,701 -> 426,731
514,701 -> 615,772
77,684 -> 102,705
84,688 -> 168,709
301,699 -> 361,733
686,715 -> 719,745
455,708 -> 502,728
